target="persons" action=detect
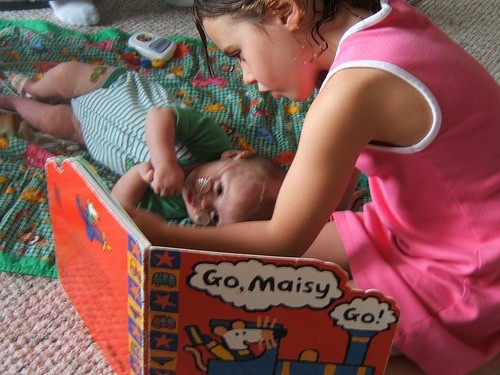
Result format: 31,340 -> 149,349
0,61 -> 287,225
110,1 -> 499,375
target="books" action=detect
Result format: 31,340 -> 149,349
46,152 -> 401,374
